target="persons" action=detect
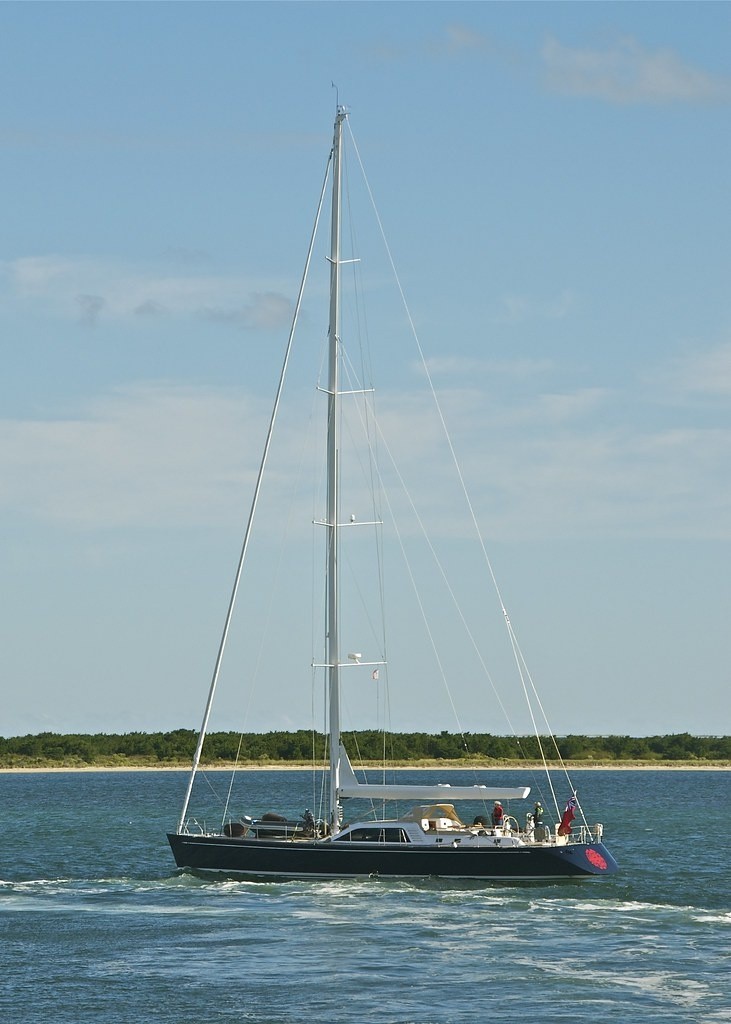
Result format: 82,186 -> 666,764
493,801 -> 503,825
531,802 -> 544,842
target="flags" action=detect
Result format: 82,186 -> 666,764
565,791 -> 578,812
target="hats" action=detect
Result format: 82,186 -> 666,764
535,802 -> 541,807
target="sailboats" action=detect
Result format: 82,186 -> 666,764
164,80 -> 623,882
